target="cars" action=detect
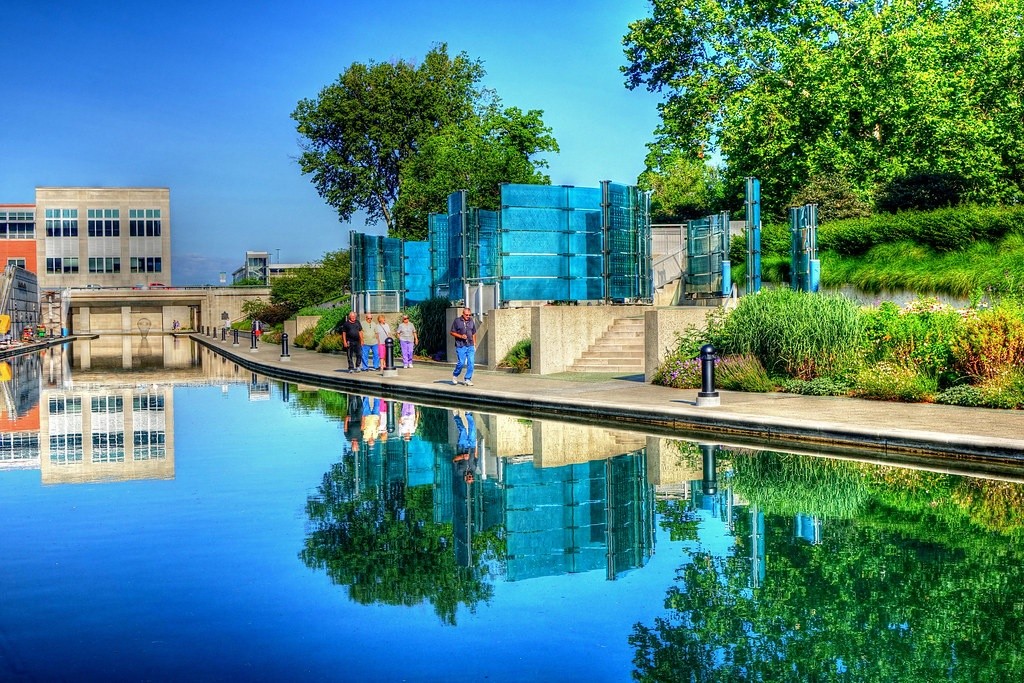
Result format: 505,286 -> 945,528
150,283 -> 175,290
85,284 -> 105,290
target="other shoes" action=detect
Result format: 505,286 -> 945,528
349,366 -> 386,374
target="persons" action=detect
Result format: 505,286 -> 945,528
251,317 -> 263,341
451,409 -> 479,485
173,320 -> 180,330
450,308 -> 477,386
342,312 -> 419,374
223,318 -> 231,336
343,394 -> 420,451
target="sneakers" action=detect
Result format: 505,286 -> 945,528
462,379 -> 474,386
404,365 -> 408,368
409,364 -> 413,368
452,371 -> 457,385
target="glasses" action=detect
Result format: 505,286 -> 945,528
404,317 -> 409,320
464,313 -> 471,316
368,317 -> 372,320
